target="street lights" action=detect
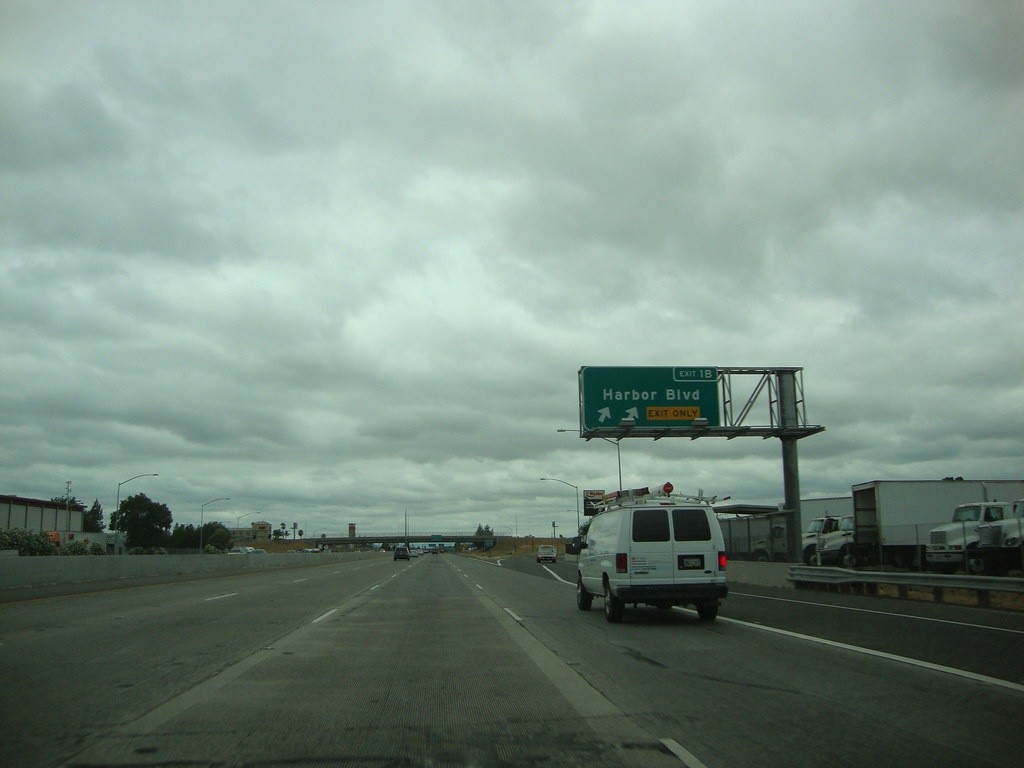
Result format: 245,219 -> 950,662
557,429 -> 622,490
238,511 -> 261,529
115,473 -> 160,555
199,497 -> 231,554
540,478 -> 581,537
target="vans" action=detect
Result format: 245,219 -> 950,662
577,482 -> 729,623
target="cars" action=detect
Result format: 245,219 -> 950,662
537,544 -> 558,564
410,547 -> 448,558
227,546 -> 266,554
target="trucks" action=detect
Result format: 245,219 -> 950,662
799,500 -> 1024,577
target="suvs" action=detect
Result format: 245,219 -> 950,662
393,546 -> 411,562
